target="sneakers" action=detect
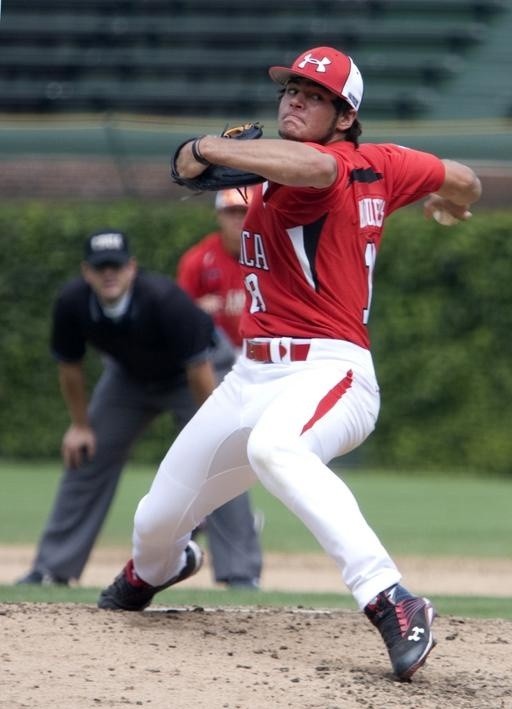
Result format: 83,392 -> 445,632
97,540 -> 204,611
364,583 -> 438,681
15,566 -> 68,588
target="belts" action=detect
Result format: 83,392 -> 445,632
246,341 -> 308,362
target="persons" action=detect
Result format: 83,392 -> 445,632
97,47 -> 483,679
177,186 -> 254,356
16,229 -> 261,590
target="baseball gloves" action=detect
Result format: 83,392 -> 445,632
169,121 -> 271,190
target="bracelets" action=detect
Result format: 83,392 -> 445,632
192,138 -> 208,166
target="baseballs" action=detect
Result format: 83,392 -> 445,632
430,205 -> 458,225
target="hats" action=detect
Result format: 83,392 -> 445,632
82,229 -> 131,267
271,45 -> 365,115
215,185 -> 253,210
